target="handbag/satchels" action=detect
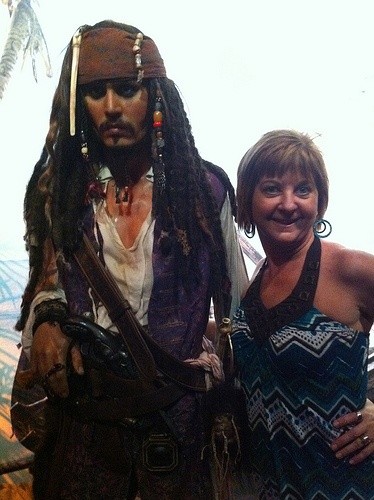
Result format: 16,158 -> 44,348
151,372 -> 252,500
12,349 -> 45,449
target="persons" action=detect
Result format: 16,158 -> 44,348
230,130 -> 374,500
17,21 -> 249,500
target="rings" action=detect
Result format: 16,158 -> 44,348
46,370 -> 56,377
53,363 -> 64,372
359,435 -> 369,444
357,410 -> 362,421
